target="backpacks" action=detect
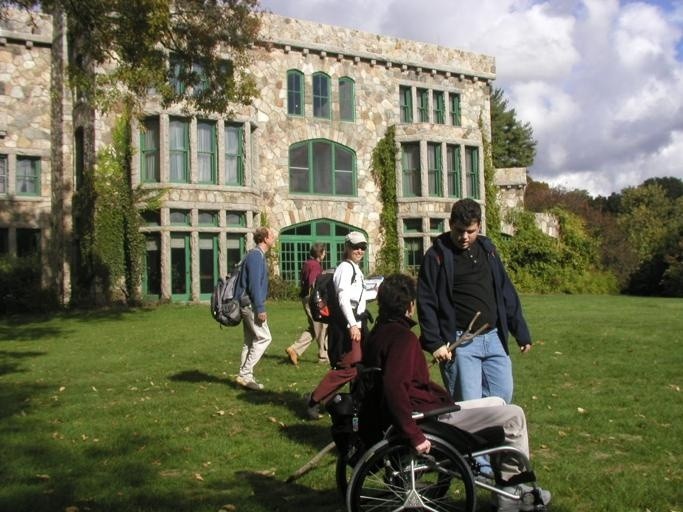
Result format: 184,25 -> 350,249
211,260 -> 242,327
308,272 -> 335,325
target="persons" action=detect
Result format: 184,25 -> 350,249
235,225 -> 275,390
303,232 -> 377,421
416,197 -> 532,480
285,243 -> 330,364
357,274 -> 551,511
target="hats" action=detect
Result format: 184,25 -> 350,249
345,231 -> 368,245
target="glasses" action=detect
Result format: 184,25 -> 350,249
350,245 -> 367,250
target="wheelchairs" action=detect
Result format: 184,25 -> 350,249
329,362 -> 541,512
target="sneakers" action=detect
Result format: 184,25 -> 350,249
498,484 -> 552,512
285,346 -> 298,366
235,375 -> 264,391
303,396 -> 321,420
